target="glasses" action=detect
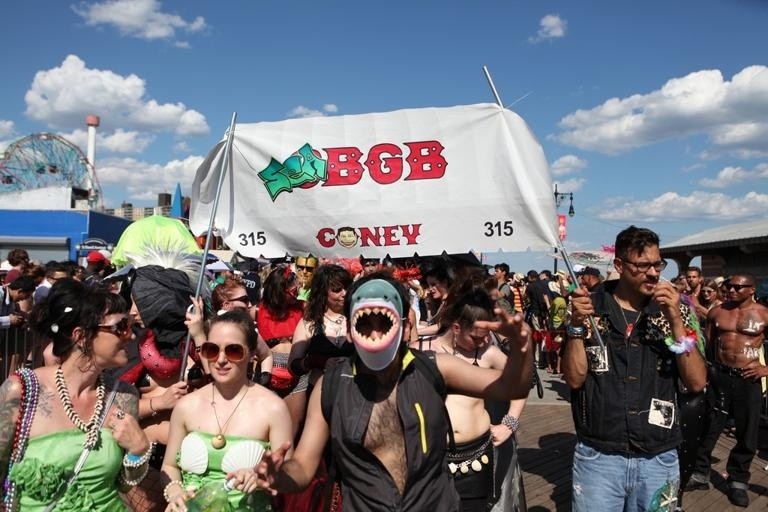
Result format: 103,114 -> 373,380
99,315 -> 129,339
200,340 -> 250,362
227,295 -> 249,303
621,257 -> 668,273
725,282 -> 754,291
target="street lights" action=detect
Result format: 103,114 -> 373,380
551,184 -> 577,276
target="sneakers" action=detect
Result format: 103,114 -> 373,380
684,472 -> 709,491
727,481 -> 749,507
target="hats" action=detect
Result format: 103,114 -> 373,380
512,272 -> 525,282
573,264 -> 601,276
547,280 -> 570,297
